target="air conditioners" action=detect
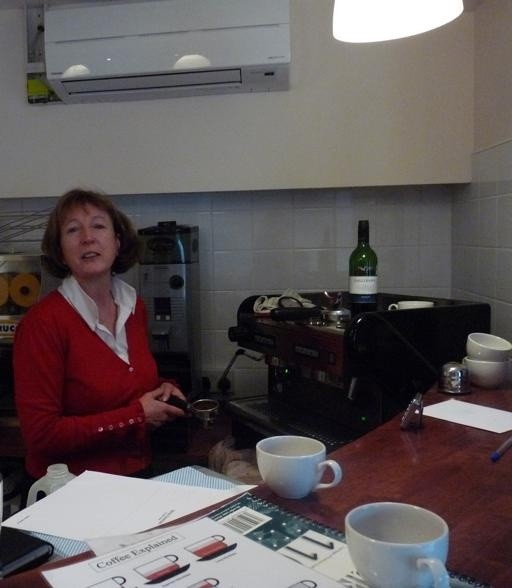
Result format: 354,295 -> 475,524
43,0 -> 291,101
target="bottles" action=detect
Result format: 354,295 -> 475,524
349,220 -> 379,318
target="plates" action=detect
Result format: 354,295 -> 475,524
145,563 -> 191,584
197,542 -> 237,562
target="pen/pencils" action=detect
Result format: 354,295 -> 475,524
491,437 -> 512,462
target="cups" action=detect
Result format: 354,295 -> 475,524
133,554 -> 180,581
183,534 -> 228,559
345,502 -> 450,588
388,301 -> 435,311
256,434 -> 343,500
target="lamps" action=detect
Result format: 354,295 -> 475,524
332,0 -> 464,44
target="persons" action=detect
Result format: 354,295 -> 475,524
11,188 -> 191,492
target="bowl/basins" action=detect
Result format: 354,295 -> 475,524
463,333 -> 512,389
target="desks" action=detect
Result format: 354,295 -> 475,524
1,381 -> 512,588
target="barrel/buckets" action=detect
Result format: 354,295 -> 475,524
26,464 -> 78,507
